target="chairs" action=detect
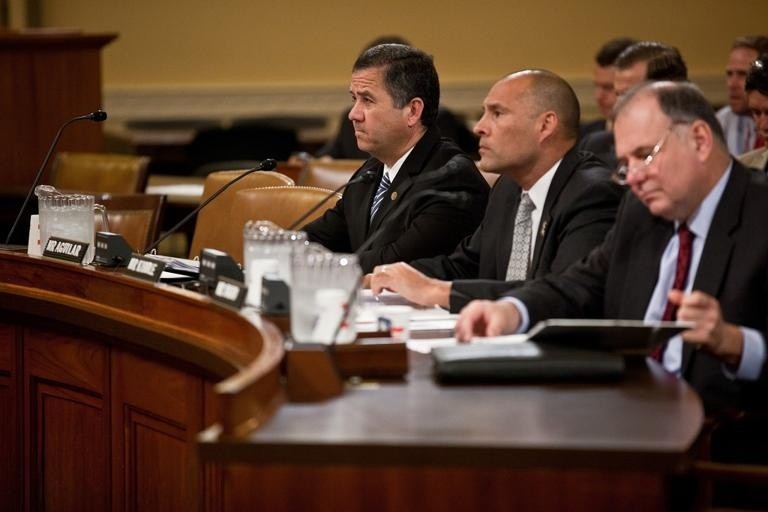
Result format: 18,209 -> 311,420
49,152 -> 154,192
86,190 -> 166,256
298,160 -> 368,188
231,184 -> 343,274
188,167 -> 295,277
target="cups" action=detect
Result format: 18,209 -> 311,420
377,304 -> 414,341
240,218 -> 362,345
33,191 -> 111,265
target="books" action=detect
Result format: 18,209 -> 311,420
471,317 -> 695,356
430,343 -> 628,384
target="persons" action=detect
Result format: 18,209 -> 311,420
360,68 -> 614,314
735,56 -> 766,171
328,33 -> 479,160
580,38 -> 635,139
451,80 -> 766,467
714,36 -> 768,158
293,43 -> 490,277
574,39 -> 688,169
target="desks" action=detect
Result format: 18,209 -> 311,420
1,245 -> 707,511
146,175 -> 206,257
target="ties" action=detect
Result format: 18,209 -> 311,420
369,172 -> 390,222
650,221 -> 695,363
505,195 -> 536,281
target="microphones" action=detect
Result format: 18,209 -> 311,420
97,158 -> 277,265
262,187 -> 472,316
200,170 -> 376,290
4,110 -> 107,244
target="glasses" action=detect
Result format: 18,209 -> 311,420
611,120 -> 687,186
